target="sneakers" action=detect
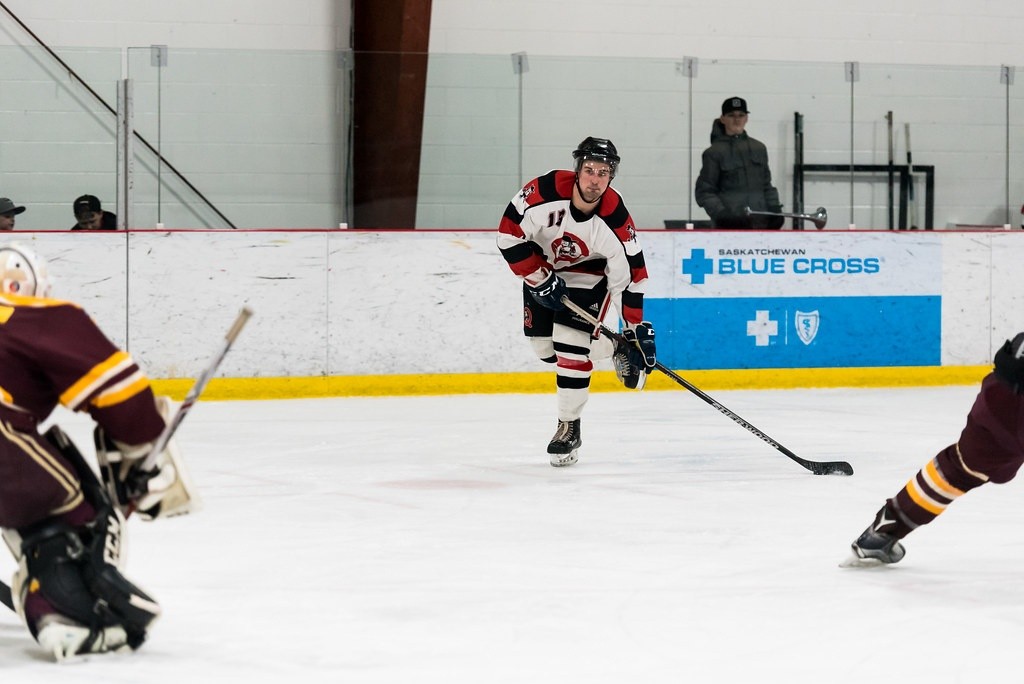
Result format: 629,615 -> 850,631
547,417 -> 582,466
612,333 -> 646,390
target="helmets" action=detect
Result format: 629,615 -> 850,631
572,136 -> 620,167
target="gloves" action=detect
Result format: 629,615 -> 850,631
524,267 -> 569,312
620,321 -> 657,374
114,441 -> 175,520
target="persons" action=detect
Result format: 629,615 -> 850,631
695,97 -> 784,230
851,333 -> 1024,564
495,137 -> 656,454
0,197 -> 26,230
0,241 -> 177,659
70,195 -> 116,230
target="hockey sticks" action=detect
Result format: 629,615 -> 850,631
122,304 -> 255,520
561,293 -> 854,476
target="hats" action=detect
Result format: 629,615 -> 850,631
74,194 -> 101,213
722,97 -> 750,116
0,198 -> 25,215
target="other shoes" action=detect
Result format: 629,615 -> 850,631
838,499 -> 905,569
24,587 -> 132,664
